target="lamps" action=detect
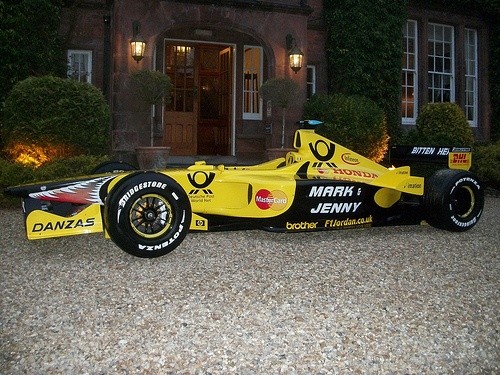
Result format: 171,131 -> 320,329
131,19 -> 148,64
287,34 -> 304,75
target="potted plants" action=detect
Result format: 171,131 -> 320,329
259,76 -> 303,165
135,69 -> 174,170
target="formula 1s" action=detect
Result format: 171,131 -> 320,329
21,119 -> 486,259
